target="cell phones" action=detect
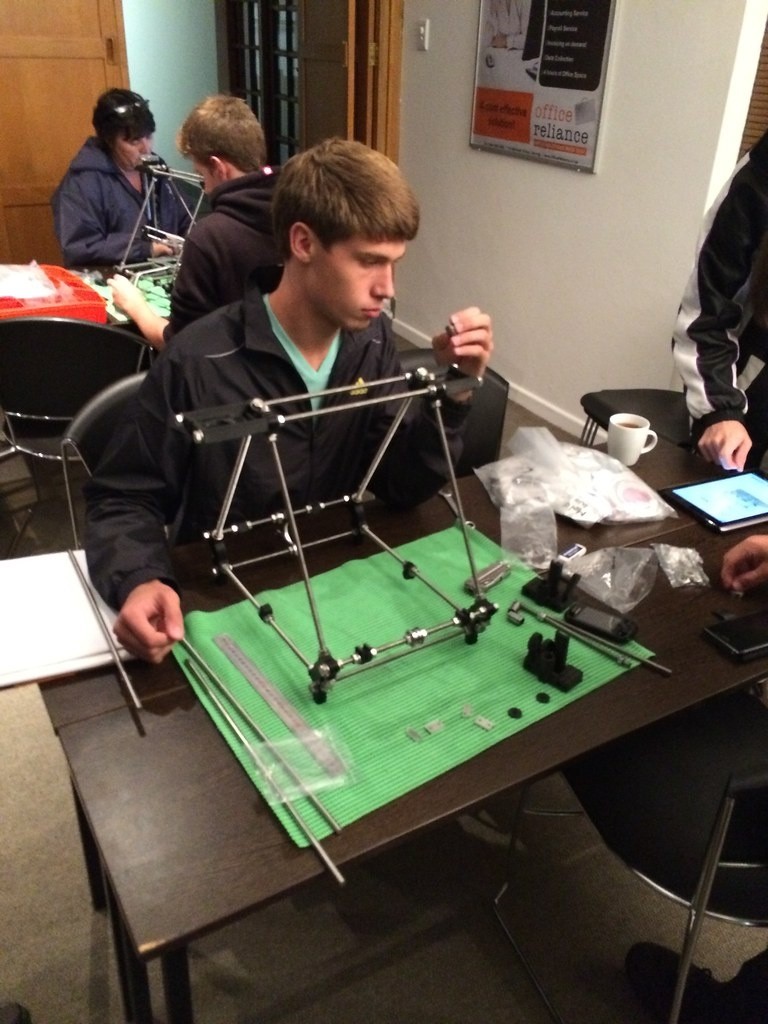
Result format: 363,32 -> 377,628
564,602 -> 638,644
705,611 -> 768,661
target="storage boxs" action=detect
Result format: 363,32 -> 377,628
0,264 -> 106,326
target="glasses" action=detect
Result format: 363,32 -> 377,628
98,99 -> 149,127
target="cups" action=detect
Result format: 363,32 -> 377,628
607,413 -> 658,468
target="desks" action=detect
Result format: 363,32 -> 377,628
36,431 -> 767,1024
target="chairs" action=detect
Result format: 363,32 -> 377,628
59,369 -> 154,550
394,347 -> 510,479
575,385 -> 694,450
489,692 -> 767,1024
0,317 -> 160,560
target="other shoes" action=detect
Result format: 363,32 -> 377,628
625,940 -> 730,1024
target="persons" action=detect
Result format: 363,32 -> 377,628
672,129 -> 767,473
50,88 -> 197,281
626,943 -> 768,1024
83,140 -> 493,664
480,0 -> 530,56
107,94 -> 285,352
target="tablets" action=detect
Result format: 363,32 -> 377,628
660,467 -> 768,526
